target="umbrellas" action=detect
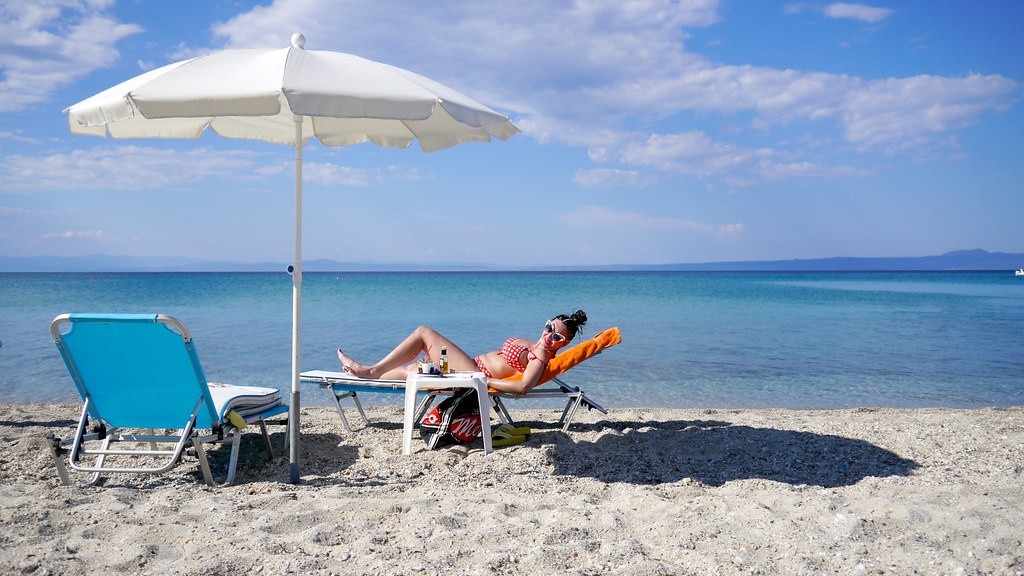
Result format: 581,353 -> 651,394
63,48 -> 521,481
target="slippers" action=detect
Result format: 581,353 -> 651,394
497,424 -> 531,438
491,429 -> 526,446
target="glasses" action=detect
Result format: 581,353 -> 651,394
545,319 -> 569,342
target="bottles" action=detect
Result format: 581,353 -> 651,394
439,346 -> 449,374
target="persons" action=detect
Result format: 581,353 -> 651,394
337,310 -> 588,394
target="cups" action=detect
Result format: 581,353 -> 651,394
422,355 -> 430,363
429,361 -> 437,371
422,363 -> 430,373
417,359 -> 425,373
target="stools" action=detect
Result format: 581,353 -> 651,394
403,372 -> 493,456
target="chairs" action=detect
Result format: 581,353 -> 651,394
47,313 -> 289,487
299,326 -> 622,439
